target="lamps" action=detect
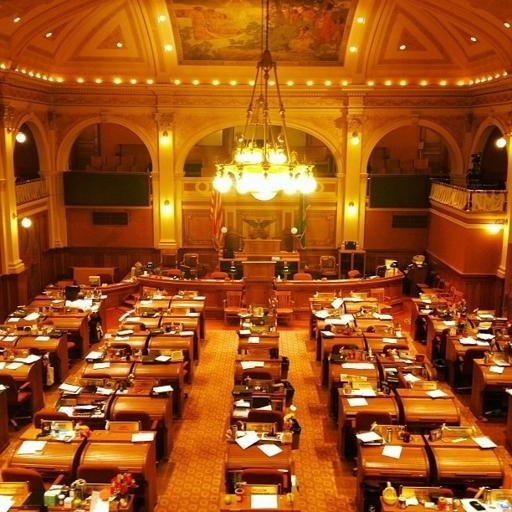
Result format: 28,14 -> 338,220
6,128 -> 25,142
348,202 -> 356,215
12,214 -> 31,227
211,0 -> 317,200
163,199 -> 170,210
495,131 -> 512,149
352,130 -> 359,144
162,130 -> 169,143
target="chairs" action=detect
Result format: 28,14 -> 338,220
293,273 -> 311,281
348,271 -> 361,278
241,470 -> 285,495
121,322 -> 145,332
246,344 -> 269,356
247,411 -> 283,433
114,412 -> 159,434
223,291 -> 244,326
351,410 -> 393,434
34,412 -> 68,430
211,272 -> 229,279
162,322 -> 184,331
370,289 -> 392,312
1,468 -> 65,502
272,292 -> 293,324
242,368 -> 272,386
77,465 -> 121,486
130,376 -> 160,389
250,396 -> 271,408
83,374 -> 111,385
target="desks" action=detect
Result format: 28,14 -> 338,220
354,433 -> 431,511
339,388 -> 400,457
445,334 -> 492,388
128,266 -> 405,317
7,440 -> 84,472
122,317 -> 159,328
148,333 -> 195,384
230,408 -> 300,450
1,286 -> 106,358
78,442 -> 158,509
160,315 -> 200,359
412,298 -> 465,340
132,361 -> 184,417
103,336 -> 148,350
309,297 -> 436,381
169,299 -> 206,339
396,388 -> 462,431
81,362 -> 132,383
427,315 -> 447,361
471,358 -> 512,420
234,356 -> 289,383
238,333 -> 279,355
0,364 -> 43,419
424,435 -> 505,488
111,396 -> 176,460
18,335 -> 69,382
135,299 -> 169,313
232,380 -> 295,414
225,444 -> 292,492
